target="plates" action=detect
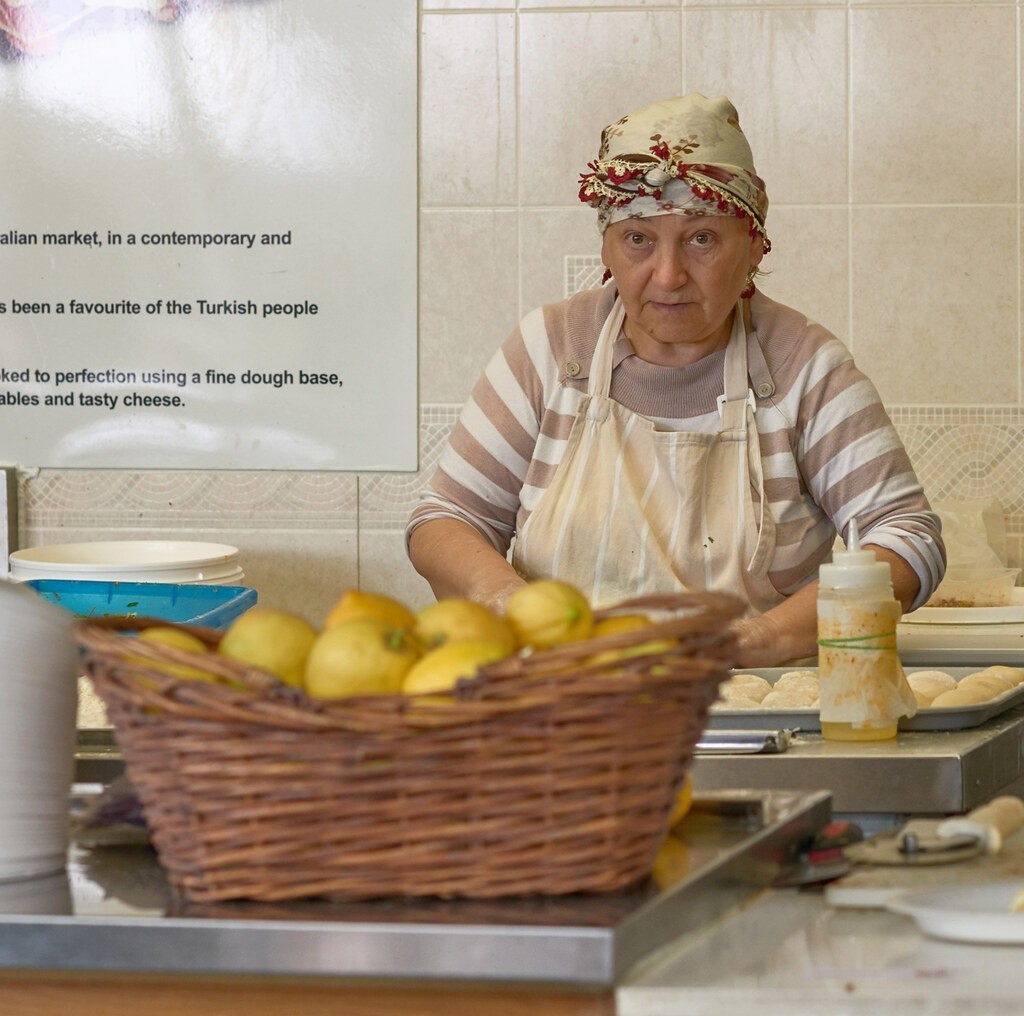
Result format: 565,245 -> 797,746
885,881 -> 1022,943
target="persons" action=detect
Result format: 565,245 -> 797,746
406,92 -> 948,670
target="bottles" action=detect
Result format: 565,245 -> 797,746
819,517 -> 900,740
0,582 -> 76,885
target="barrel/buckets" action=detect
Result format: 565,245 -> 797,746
11,537 -> 246,585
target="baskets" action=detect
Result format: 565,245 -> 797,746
72,590 -> 745,904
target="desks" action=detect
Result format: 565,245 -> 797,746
0,715 -> 1024,1016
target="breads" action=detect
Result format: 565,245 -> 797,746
703,665 -> 1024,709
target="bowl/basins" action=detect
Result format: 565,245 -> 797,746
926,567 -> 1021,606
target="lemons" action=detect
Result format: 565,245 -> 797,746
669,771 -> 696,829
122,576 -> 677,711
